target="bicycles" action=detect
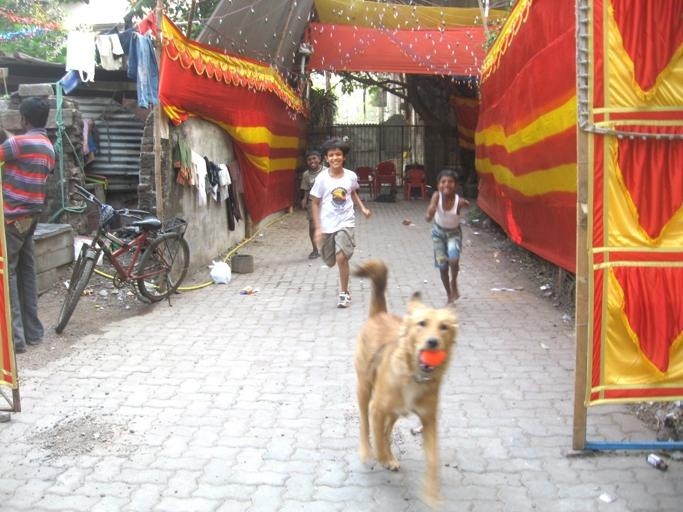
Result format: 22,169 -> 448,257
54,184 -> 190,333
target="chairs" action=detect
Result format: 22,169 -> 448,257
355,162 -> 427,201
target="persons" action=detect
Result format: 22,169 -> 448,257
300,149 -> 328,259
424,169 -> 471,305
309,138 -> 372,309
0,97 -> 55,353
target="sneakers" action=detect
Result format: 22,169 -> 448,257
336,290 -> 353,309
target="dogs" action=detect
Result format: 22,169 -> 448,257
349,259 -> 460,510
